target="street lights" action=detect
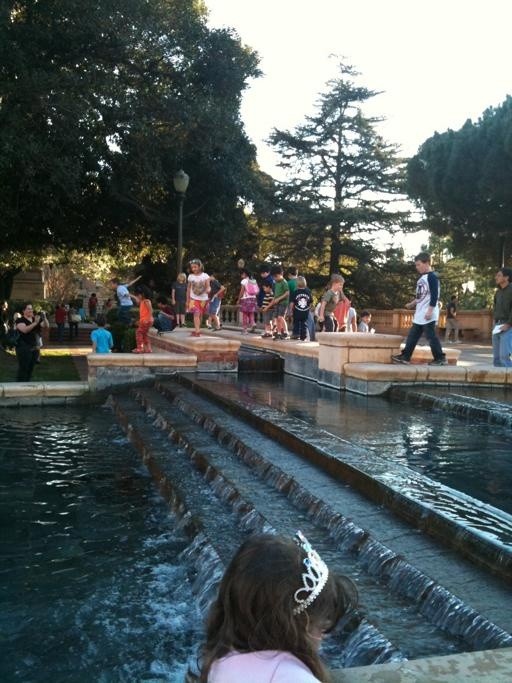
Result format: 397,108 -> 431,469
167,166 -> 193,285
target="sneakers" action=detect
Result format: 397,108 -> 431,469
428,358 -> 448,365
132,348 -> 143,353
392,354 -> 410,365
158,323 -> 318,341
144,349 -> 152,353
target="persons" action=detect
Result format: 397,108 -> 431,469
237,264 -> 371,341
493,268 -> 512,367
0,300 -> 86,381
391,252 -> 462,365
88,276 -> 177,353
183,528 -> 360,683
172,259 -> 225,336
